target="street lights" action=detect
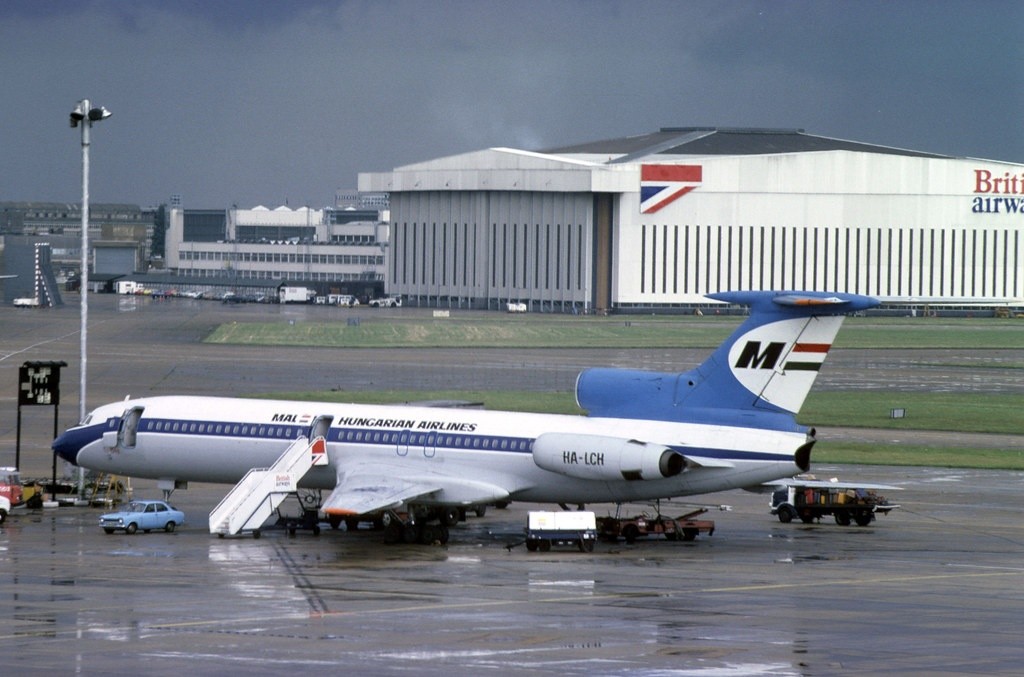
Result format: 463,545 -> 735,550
69,94 -> 109,418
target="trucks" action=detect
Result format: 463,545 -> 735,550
768,485 -> 901,527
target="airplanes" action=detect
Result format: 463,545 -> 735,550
49,290 -> 908,537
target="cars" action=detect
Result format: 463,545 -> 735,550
116,280 -> 402,309
97,499 -> 186,535
